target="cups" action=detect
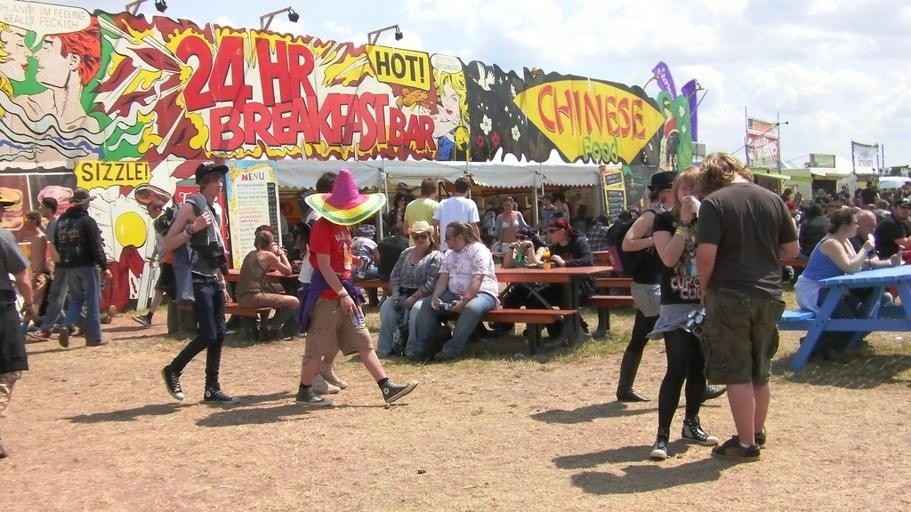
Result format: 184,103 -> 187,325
18,242 -> 33,281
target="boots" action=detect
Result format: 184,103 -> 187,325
616,351 -> 651,401
380,379 -> 419,403
682,414 -> 718,446
650,425 -> 670,459
701,385 -> 726,403
295,362 -> 347,406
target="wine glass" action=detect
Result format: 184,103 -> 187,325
866,249 -> 875,270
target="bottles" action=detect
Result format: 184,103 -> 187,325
544,244 -> 551,268
358,258 -> 367,278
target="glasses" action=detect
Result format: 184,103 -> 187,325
411,235 -> 427,240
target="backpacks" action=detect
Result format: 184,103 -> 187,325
607,208 -> 657,276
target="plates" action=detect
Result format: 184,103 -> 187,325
527,263 -> 556,269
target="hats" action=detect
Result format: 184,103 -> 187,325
305,169 -> 387,225
647,171 -> 677,190
195,161 -> 228,183
72,190 -> 96,205
895,198 -> 911,209
0,193 -> 14,207
407,220 -> 434,237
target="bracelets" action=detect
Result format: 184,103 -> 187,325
24,301 -> 33,307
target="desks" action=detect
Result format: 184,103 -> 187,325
495,266 -> 613,348
223,269 -> 300,296
779,264 -> 910,371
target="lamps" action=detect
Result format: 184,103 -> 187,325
126,0 -> 167,16
368,25 -> 403,45
261,7 -> 299,33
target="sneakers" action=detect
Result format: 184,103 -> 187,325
432,352 -> 446,361
407,351 -> 425,362
711,435 -> 760,460
204,385 -> 240,404
27,327 -> 108,347
755,427 -> 767,449
133,316 -> 151,327
161,366 -> 184,401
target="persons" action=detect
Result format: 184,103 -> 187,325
0,22 -> 34,119
780,182 -> 911,359
0,15 -> 104,169
350,176 -> 630,360
21,273 -> 50,331
658,97 -> 681,172
51,186 -> 115,348
0,195 -> 39,459
131,162 -> 306,404
25,197 -> 88,339
25,209 -> 52,335
429,68 -> 473,160
617,153 -> 800,459
297,169 -> 418,405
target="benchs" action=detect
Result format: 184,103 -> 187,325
593,277 -> 634,294
352,277 -> 390,304
777,307 -> 815,331
172,302 -> 271,345
438,308 -> 578,360
579,295 -> 634,339
